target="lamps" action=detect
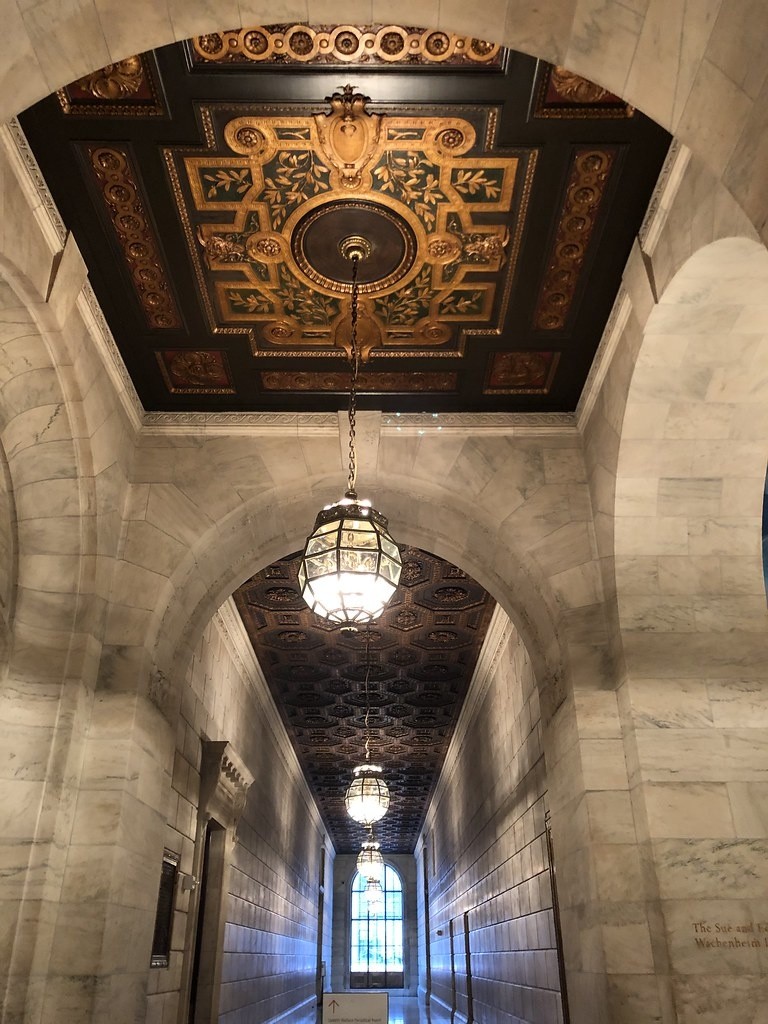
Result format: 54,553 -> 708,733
296,229 -> 406,634
345,623 -> 393,828
363,875 -> 387,916
355,823 -> 383,877
